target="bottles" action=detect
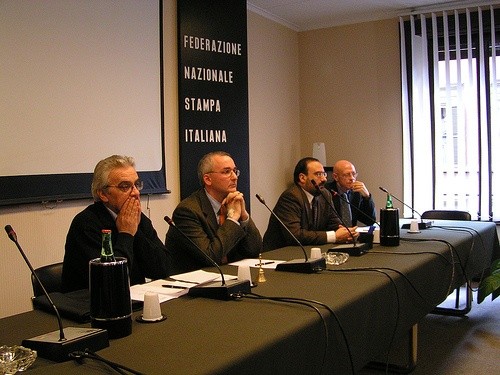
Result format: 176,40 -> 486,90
99,230 -> 116,263
386,193 -> 393,209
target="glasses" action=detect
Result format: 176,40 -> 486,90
342,173 -> 358,178
104,179 -> 144,192
317,172 -> 327,177
207,169 -> 240,178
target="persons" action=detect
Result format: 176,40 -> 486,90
165,151 -> 263,274
263,157 -> 360,253
61,155 -> 176,295
324,159 -> 376,228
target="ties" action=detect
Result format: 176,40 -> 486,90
341,193 -> 352,227
220,205 -> 229,263
312,196 -> 318,231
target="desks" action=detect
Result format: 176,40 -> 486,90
0,218 -> 500,375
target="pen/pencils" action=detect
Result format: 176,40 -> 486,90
162,285 -> 188,289
255,261 -> 274,266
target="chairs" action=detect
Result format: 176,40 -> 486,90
421,210 -> 471,307
29,262 -> 64,296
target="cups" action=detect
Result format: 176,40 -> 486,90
236,265 -> 254,286
141,292 -> 163,320
374,228 -> 380,244
410,221 -> 419,231
311,247 -> 322,259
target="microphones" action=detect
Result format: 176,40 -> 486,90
256,193 -> 326,274
330,189 -> 380,227
311,179 -> 370,256
163,216 -> 251,301
4,224 -> 109,362
379,186 -> 431,229
